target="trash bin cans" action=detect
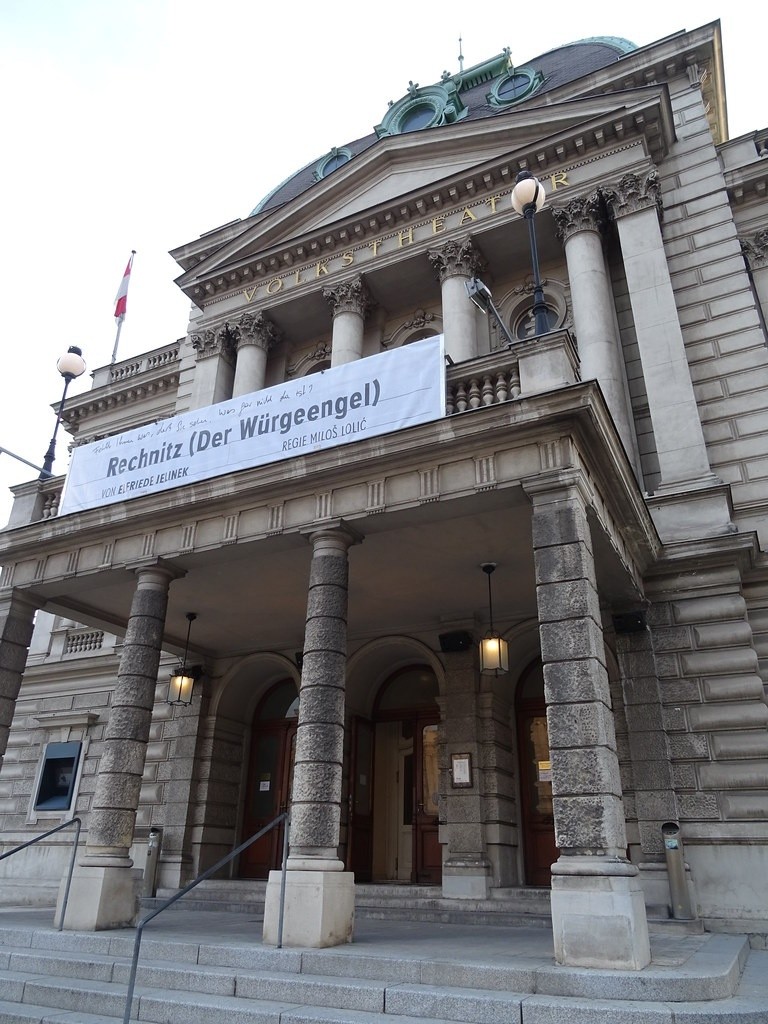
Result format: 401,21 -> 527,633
143,826 -> 163,898
660,822 -> 693,919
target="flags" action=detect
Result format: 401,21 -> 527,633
113,250 -> 136,326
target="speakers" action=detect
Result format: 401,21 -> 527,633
295,651 -> 303,669
439,630 -> 471,652
613,610 -> 647,635
174,664 -> 203,681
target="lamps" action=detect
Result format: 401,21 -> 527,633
165,612 -> 196,707
38,345 -> 86,478
438,630 -> 475,652
463,277 -> 512,343
612,610 -> 647,634
480,562 -> 509,678
510,170 -> 555,337
